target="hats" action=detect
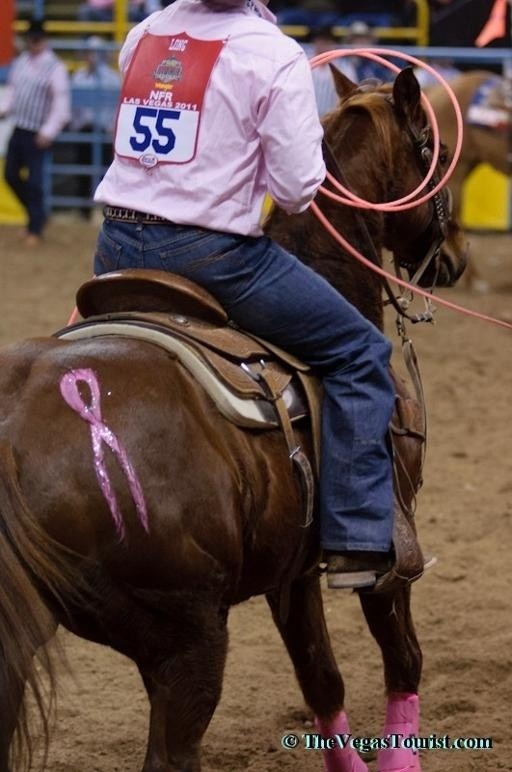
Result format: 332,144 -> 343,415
15,26 -> 54,38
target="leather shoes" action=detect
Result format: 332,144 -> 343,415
324,548 -> 438,589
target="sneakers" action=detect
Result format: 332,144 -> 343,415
25,235 -> 43,246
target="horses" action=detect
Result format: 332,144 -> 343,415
0,57 -> 473,771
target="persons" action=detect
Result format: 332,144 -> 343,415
0,0 -> 469,249
93,0 -> 397,590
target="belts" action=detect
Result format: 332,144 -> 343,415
102,205 -> 172,225
